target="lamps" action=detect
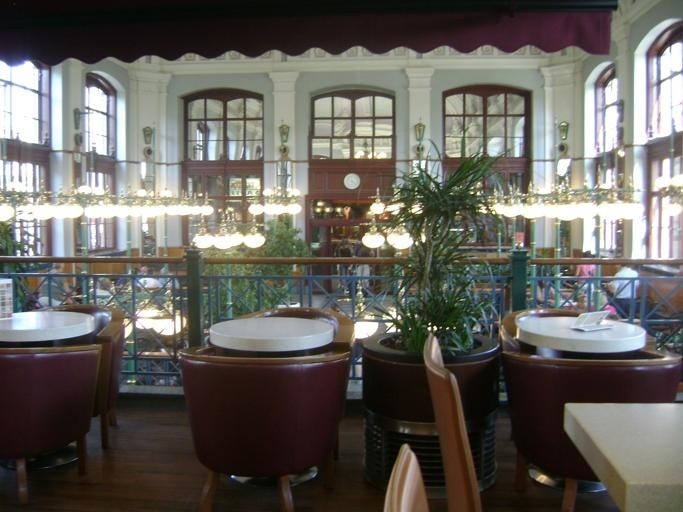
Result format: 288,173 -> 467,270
359,177 -> 648,250
0,187 -> 302,252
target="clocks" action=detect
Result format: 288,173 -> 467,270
343,172 -> 361,189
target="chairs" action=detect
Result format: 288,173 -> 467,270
1,305 -> 127,507
500,305 -> 582,361
501,348 -> 682,511
180,304 -> 354,512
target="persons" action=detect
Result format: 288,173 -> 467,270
575,251 -> 597,304
609,264 -> 640,317
90,278 -> 112,295
334,236 -> 371,289
129,253 -> 181,289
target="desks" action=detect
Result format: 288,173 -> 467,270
517,315 -> 648,359
562,402 -> 682,511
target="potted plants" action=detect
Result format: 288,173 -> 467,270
360,129 -> 540,503
